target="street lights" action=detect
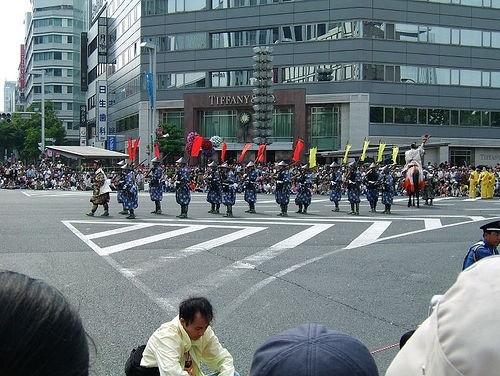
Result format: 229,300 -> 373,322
27,70 -> 44,151
139,42 -> 156,157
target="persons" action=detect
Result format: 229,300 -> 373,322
85,160 -> 112,217
403,139 -> 427,182
385,220 -> 500,376
149,157 -> 165,215
116,160 -> 138,219
0,270 -> 90,376
248,322 -> 380,376
275,160 -> 312,217
203,160 -> 259,217
0,156 -> 500,200
139,297 -> 240,376
422,169 -> 436,206
322,162 -> 394,215
173,157 -> 191,218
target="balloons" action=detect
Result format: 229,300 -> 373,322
185,131 -> 223,157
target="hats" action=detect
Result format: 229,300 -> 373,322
246,161 -> 255,167
480,220 -> 500,232
176,157 -> 186,163
278,161 -> 287,166
249,323 -> 379,376
302,164 -> 309,170
150,157 -> 159,162
116,160 -> 130,170
207,161 -> 230,168
94,159 -> 101,164
388,254 -> 500,376
330,162 -> 391,172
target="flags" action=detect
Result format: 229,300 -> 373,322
391,147 -> 399,165
359,140 -> 371,161
342,143 -> 352,164
190,135 -> 203,157
376,142 -> 386,162
291,139 -> 317,169
257,144 -> 266,163
127,136 -> 140,159
239,143 -> 251,161
221,143 -> 227,163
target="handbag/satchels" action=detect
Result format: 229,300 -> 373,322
99,179 -> 112,195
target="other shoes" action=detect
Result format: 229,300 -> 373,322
207,204 -> 220,214
86,212 -> 109,217
119,210 -> 135,219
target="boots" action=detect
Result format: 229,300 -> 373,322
177,205 -> 188,218
350,202 -> 359,215
295,204 -> 309,215
383,204 -> 391,214
424,200 -> 433,206
368,201 -> 377,212
150,201 -> 162,214
331,201 -> 340,212
224,205 -> 235,218
277,205 -> 289,217
245,203 -> 256,214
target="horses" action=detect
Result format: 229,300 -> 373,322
405,165 -> 426,207
